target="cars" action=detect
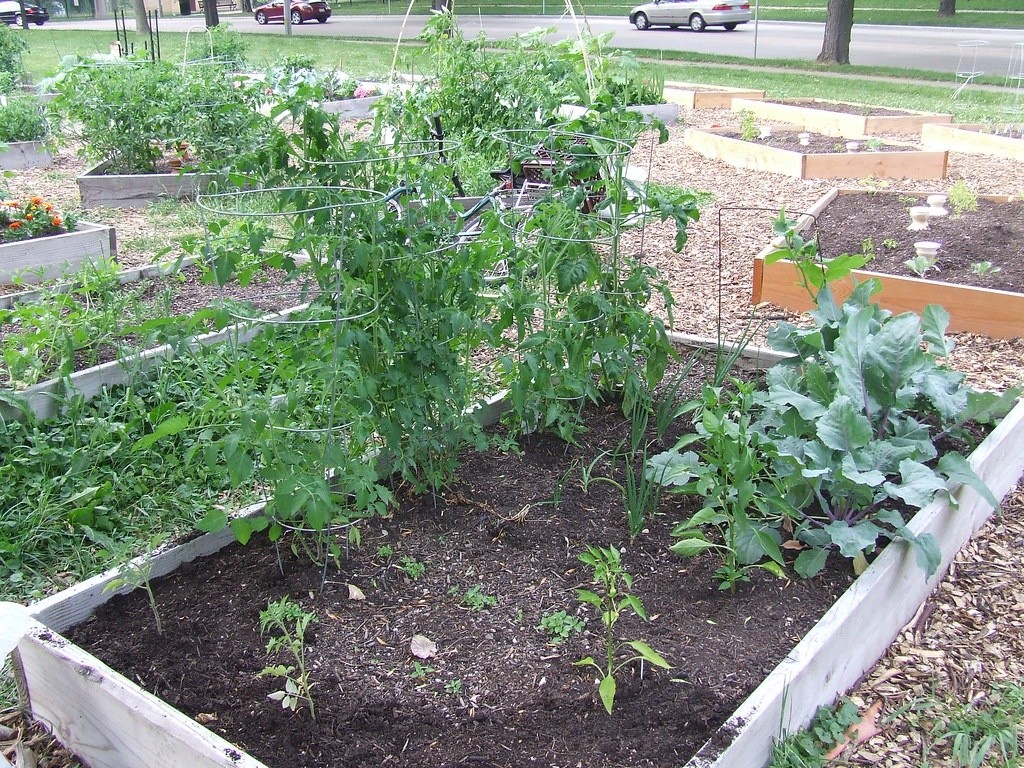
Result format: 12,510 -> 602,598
253,0 -> 332,25
0,0 -> 51,26
628,0 -> 751,31
